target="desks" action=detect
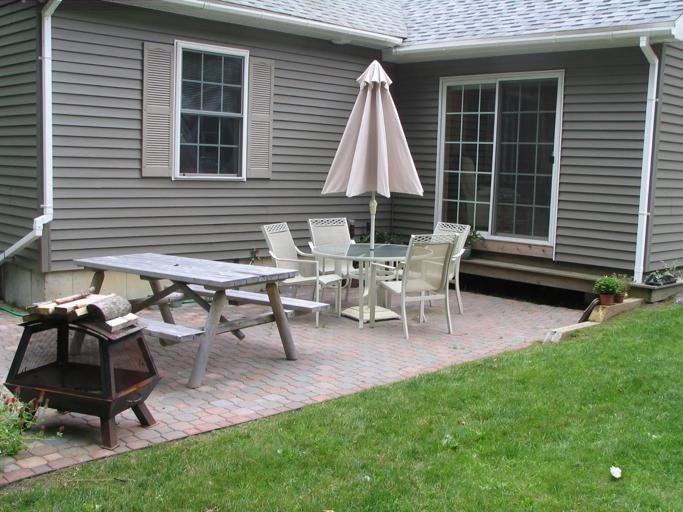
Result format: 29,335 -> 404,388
72,253 -> 299,390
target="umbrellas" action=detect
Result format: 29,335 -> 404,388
321,60 -> 424,306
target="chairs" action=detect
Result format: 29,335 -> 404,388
262,217 -> 471,339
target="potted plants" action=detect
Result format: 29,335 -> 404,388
594,272 -> 631,303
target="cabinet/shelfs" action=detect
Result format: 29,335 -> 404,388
3,316 -> 159,449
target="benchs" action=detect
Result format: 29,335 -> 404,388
40,290 -> 330,389
